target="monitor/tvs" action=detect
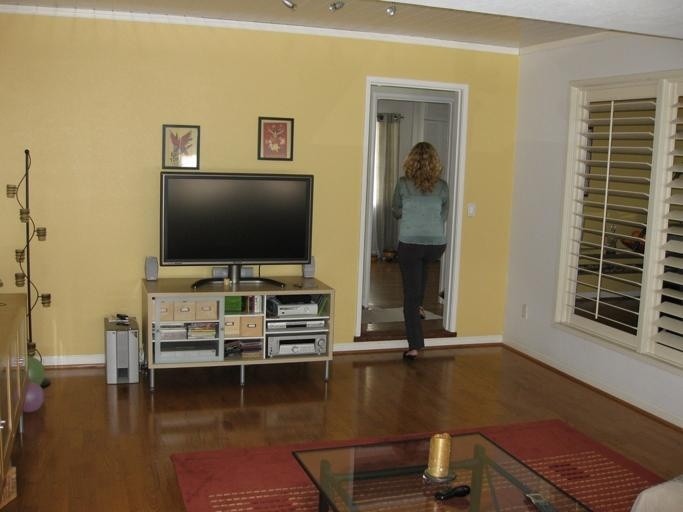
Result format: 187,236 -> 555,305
160,172 -> 314,288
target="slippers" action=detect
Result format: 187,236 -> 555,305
402,349 -> 416,364
416,307 -> 425,319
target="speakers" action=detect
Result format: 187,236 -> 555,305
212,267 -> 253,279
302,256 -> 315,278
145,257 -> 158,281
104,318 -> 139,384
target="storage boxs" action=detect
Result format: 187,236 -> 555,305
173,299 -> 196,321
224,316 -> 241,337
196,300 -> 218,320
240,317 -> 263,337
152,299 -> 173,321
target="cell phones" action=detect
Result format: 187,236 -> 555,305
526,493 -> 556,512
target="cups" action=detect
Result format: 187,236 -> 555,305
428,434 -> 450,477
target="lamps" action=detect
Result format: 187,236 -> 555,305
384,3 -> 396,16
283,0 -> 296,9
6,149 -> 53,387
329,1 -> 344,12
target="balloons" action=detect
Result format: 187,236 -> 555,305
28,356 -> 45,385
23,383 -> 44,413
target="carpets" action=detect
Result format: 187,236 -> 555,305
171,420 -> 642,511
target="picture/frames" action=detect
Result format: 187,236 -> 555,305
162,124 -> 200,169
258,117 -> 294,160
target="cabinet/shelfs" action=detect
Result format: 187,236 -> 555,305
0,293 -> 29,512
142,276 -> 336,390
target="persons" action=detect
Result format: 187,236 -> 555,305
390,140 -> 450,359
